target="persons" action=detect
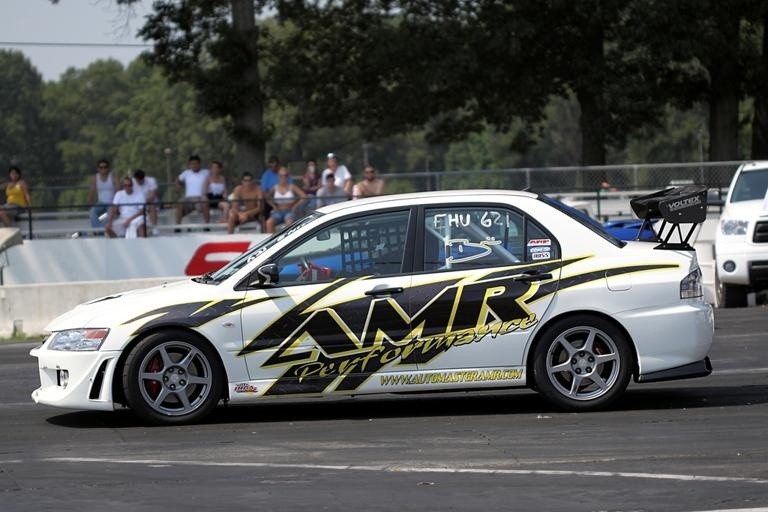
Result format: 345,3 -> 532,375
227,151 -> 386,235
86,158 -> 123,235
200,160 -> 230,232
298,222 -> 406,282
175,155 -> 209,232
1,167 -> 33,227
104,176 -> 147,237
132,169 -> 161,236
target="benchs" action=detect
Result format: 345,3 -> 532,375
14,211 -> 285,239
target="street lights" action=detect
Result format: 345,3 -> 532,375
163,146 -> 173,209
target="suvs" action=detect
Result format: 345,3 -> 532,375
713,156 -> 767,308
